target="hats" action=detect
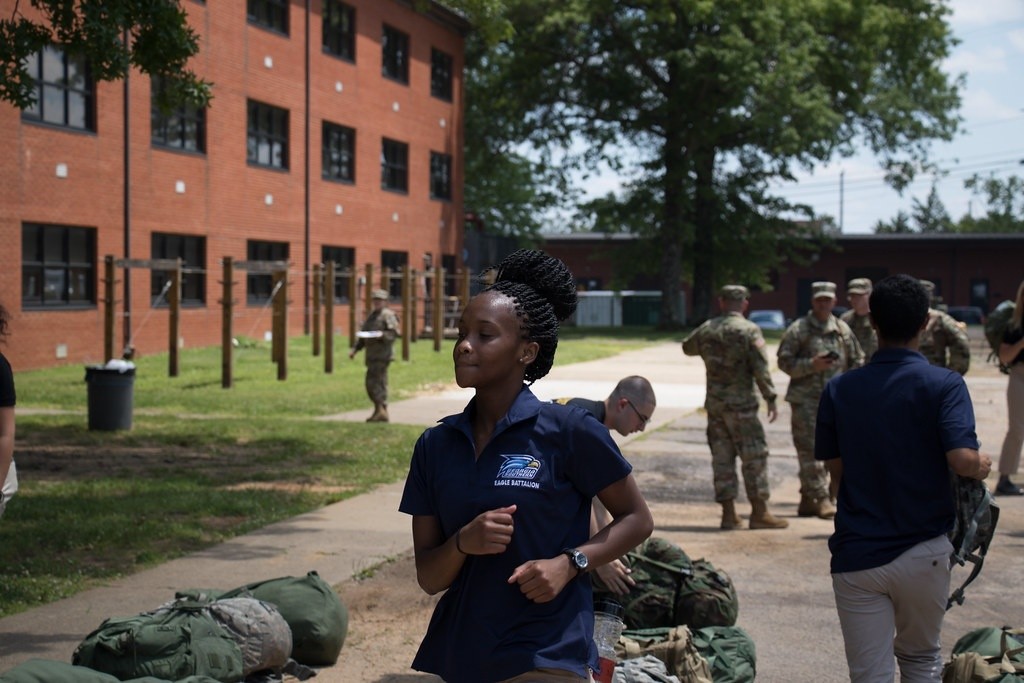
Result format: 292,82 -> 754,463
811,282 -> 837,300
719,284 -> 750,299
372,289 -> 388,300
846,278 -> 873,294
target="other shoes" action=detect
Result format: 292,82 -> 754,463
798,493 -> 837,518
994,480 -> 1023,496
367,406 -> 390,421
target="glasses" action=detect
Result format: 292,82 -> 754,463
621,397 -> 651,426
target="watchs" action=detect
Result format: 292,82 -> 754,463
561,548 -> 588,578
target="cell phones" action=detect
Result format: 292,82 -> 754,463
823,350 -> 840,363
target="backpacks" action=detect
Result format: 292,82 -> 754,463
947,462 -> 1000,610
984,300 -> 1017,375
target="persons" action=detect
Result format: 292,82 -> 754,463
994,280 -> 1024,497
349,289 -> 401,421
0,304 -> 18,517
814,276 -> 992,683
399,248 -> 656,683
776,278 -> 971,517
682,287 -> 790,529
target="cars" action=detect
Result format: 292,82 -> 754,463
745,308 -> 784,329
947,307 -> 981,327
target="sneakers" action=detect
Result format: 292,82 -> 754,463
750,504 -> 790,529
721,501 -> 742,530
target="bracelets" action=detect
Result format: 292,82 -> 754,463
456,526 -> 469,554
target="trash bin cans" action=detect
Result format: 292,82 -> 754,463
80,365 -> 140,432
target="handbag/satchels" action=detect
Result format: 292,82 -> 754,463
594,536 -> 757,683
70,568 -> 347,683
